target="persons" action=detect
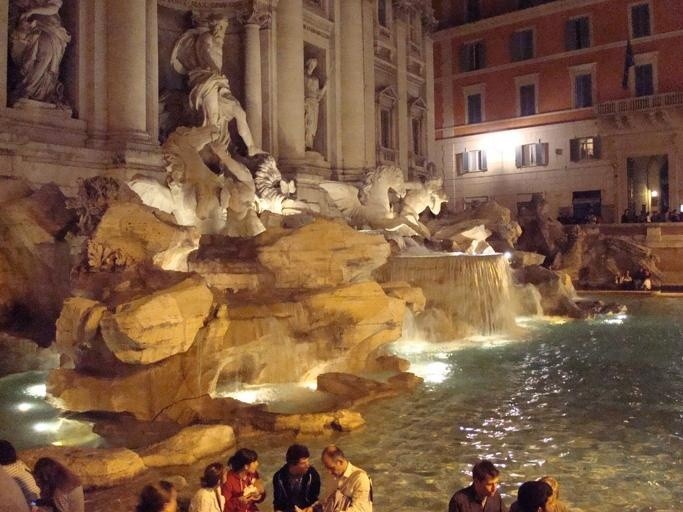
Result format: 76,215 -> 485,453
273,444 -> 323,512
508,479 -> 553,512
304,57 -> 327,151
539,476 -> 570,512
620,202 -> 683,223
32,456 -> 86,511
620,268 -> 652,291
221,448 -> 266,512
136,480 -> 178,512
187,462 -> 228,512
170,13 -> 266,156
301,446 -> 373,512
448,459 -> 508,512
0,438 -> 39,512
10,1 -> 73,101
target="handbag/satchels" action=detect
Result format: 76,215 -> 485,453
321,490 -> 350,512
